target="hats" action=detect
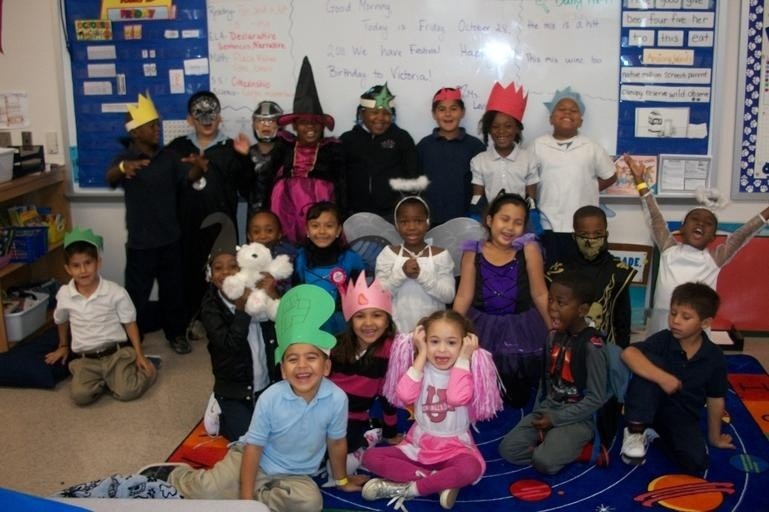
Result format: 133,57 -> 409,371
277,55 -> 334,131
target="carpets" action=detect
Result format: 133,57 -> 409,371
0,294 -> 160,393
160,346 -> 769,510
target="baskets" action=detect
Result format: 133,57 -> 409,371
0,227 -> 49,263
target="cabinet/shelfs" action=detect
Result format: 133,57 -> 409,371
1,158 -> 72,359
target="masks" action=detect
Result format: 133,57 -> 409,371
575,235 -> 607,260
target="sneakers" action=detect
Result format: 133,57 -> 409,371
619,427 -> 660,465
204,392 -> 222,438
439,488 -> 460,509
362,478 -> 414,512
144,355 -> 162,369
595,444 -> 609,468
138,462 -> 190,482
169,320 -> 207,354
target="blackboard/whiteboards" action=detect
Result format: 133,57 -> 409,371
205,1 -> 623,158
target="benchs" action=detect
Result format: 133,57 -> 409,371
669,235 -> 769,339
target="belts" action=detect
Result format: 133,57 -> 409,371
85,345 -> 116,360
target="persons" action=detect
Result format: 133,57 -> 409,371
45,81 -> 769,511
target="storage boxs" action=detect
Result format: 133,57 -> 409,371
2,288 -> 50,341
0,147 -> 19,183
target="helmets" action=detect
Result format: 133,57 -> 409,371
252,102 -> 283,144
